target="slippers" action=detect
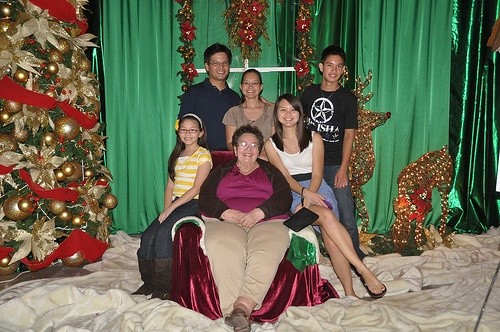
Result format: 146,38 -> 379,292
360,275 -> 387,298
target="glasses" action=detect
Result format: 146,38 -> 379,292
236,140 -> 260,149
209,61 -> 230,67
179,128 -> 198,133
242,81 -> 260,86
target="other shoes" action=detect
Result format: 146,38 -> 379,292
225,308 -> 251,332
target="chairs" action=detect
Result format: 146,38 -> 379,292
171,151 -> 340,324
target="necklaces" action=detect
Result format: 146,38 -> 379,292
320,83 -> 341,104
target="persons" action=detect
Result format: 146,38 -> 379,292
263,97 -> 389,299
300,44 -> 366,277
198,124 -> 293,332
132,113 -> 214,300
178,43 -> 275,151
221,69 -> 275,162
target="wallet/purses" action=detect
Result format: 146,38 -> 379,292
283,207 -> 319,232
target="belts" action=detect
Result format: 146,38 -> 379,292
290,173 -> 313,182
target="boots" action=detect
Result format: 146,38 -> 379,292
133,258 -> 153,295
148,257 -> 172,301
